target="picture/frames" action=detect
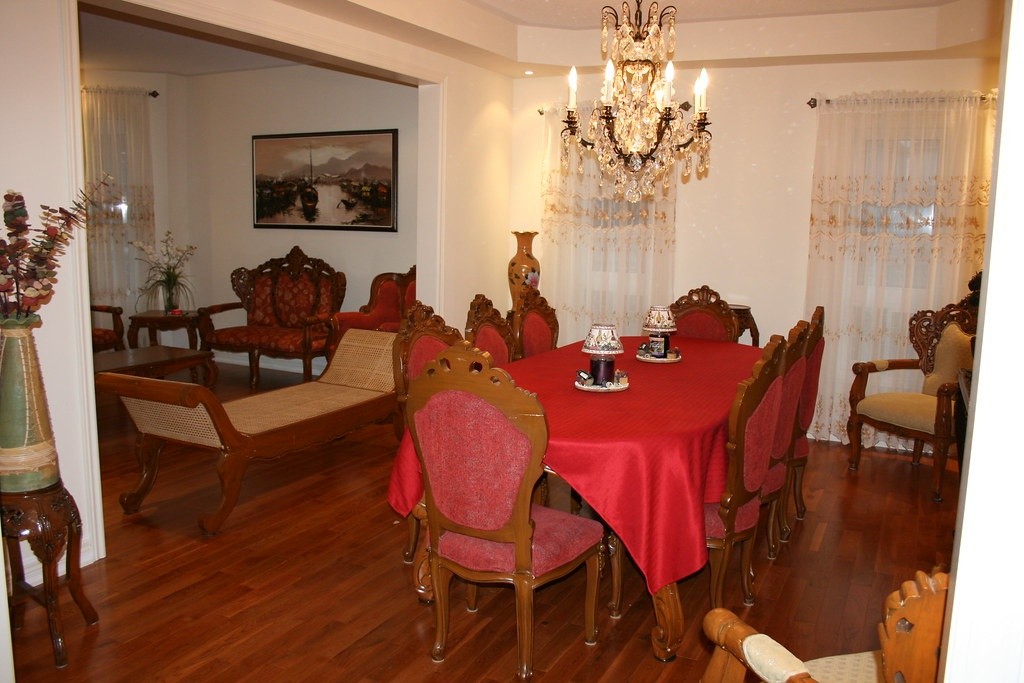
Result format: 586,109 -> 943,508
252,129 -> 399,232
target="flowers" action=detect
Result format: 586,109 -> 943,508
0,169 -> 116,318
127,229 -> 198,314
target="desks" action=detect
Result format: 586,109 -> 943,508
94,344 -> 217,392
727,303 -> 760,346
386,335 -> 823,660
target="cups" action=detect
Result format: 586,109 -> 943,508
667,353 -> 677,359
618,377 -> 628,385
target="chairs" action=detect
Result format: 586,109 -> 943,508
197,245 -> 346,389
846,271 -> 983,503
695,570 -> 950,683
406,341 -> 605,683
464,294 -> 515,363
197,246 -> 347,389
793,305 -> 825,519
669,286 -> 740,342
325,264 -> 416,362
510,285 -> 559,359
759,320 -> 810,560
392,299 -> 550,612
90,304 -> 126,353
608,334 -> 786,620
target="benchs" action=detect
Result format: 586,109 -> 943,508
94,328 -> 410,535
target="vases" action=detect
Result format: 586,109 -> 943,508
0,314 -> 60,492
507,230 -> 540,311
162,284 -> 180,315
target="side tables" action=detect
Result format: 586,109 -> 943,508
126,310 -> 199,350
0,478 -> 99,671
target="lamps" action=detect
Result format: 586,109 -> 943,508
560,0 -> 711,203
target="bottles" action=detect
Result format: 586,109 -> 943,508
674,347 -> 679,358
575,368 -> 594,385
